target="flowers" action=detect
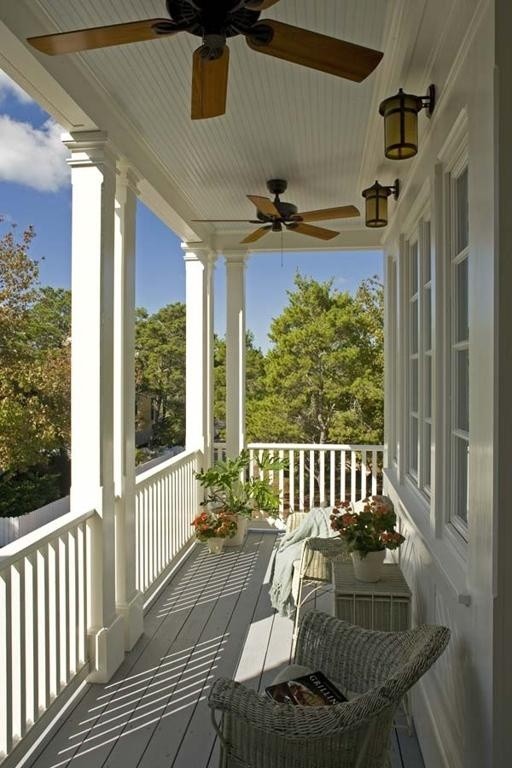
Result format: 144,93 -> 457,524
189,512 -> 238,543
329,494 -> 403,560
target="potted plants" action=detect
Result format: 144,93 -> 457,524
191,448 -> 289,546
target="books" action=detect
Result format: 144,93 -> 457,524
265,670 -> 349,707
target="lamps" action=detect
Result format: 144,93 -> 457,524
361,179 -> 400,227
378,84 -> 437,159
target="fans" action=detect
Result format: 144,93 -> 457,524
26,2 -> 385,123
190,179 -> 360,244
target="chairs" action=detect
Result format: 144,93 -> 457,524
289,536 -> 350,665
264,497 -> 378,607
206,608 -> 451,768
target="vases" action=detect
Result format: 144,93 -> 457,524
350,548 -> 387,583
206,536 -> 223,554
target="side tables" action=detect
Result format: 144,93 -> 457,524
332,561 -> 413,633
